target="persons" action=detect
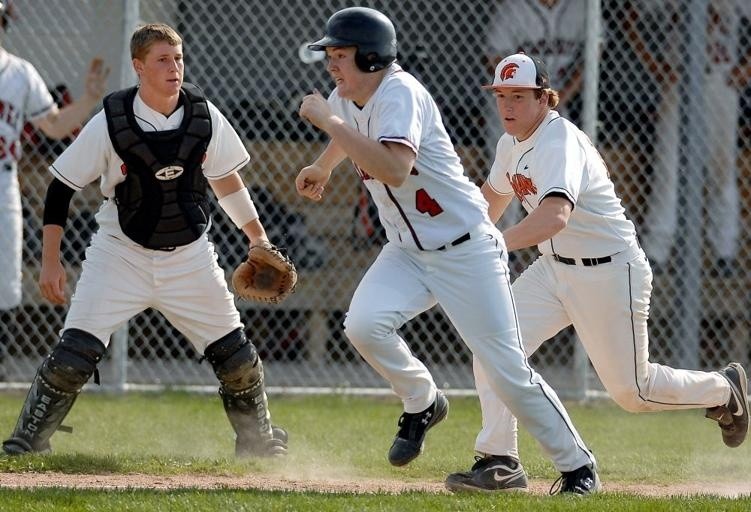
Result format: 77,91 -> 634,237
486,0 -> 605,227
296,5 -> 604,503
0,0 -> 112,311
641,0 -> 751,267
1,22 -> 296,472
443,51 -> 750,494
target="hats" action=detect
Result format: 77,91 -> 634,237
481,51 -> 549,91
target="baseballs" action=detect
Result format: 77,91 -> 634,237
299,42 -> 321,63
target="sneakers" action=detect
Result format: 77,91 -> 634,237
387,390 -> 449,466
549,464 -> 607,500
704,360 -> 750,449
446,453 -> 529,495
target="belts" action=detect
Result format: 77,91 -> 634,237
437,232 -> 470,251
553,254 -> 612,268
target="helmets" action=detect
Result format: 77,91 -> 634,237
307,7 -> 399,73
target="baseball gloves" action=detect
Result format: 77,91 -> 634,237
230,243 -> 299,304
